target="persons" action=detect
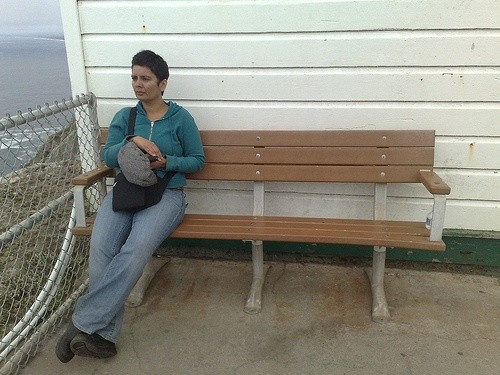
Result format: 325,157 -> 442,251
56,50 -> 205,364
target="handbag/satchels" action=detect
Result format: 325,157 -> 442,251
112,107 -> 175,212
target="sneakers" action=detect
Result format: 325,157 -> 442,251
56,321 -> 86,363
70,332 -> 117,359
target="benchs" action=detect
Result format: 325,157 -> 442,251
72,125 -> 451,323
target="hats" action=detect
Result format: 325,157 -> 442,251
118,142 -> 157,186
